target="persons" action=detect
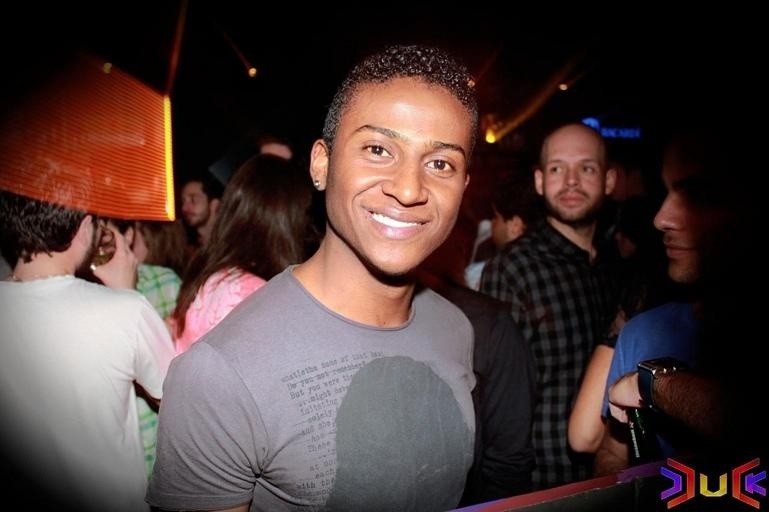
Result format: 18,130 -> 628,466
0,41 -> 769,512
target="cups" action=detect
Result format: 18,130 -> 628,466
93,229 -> 116,265
